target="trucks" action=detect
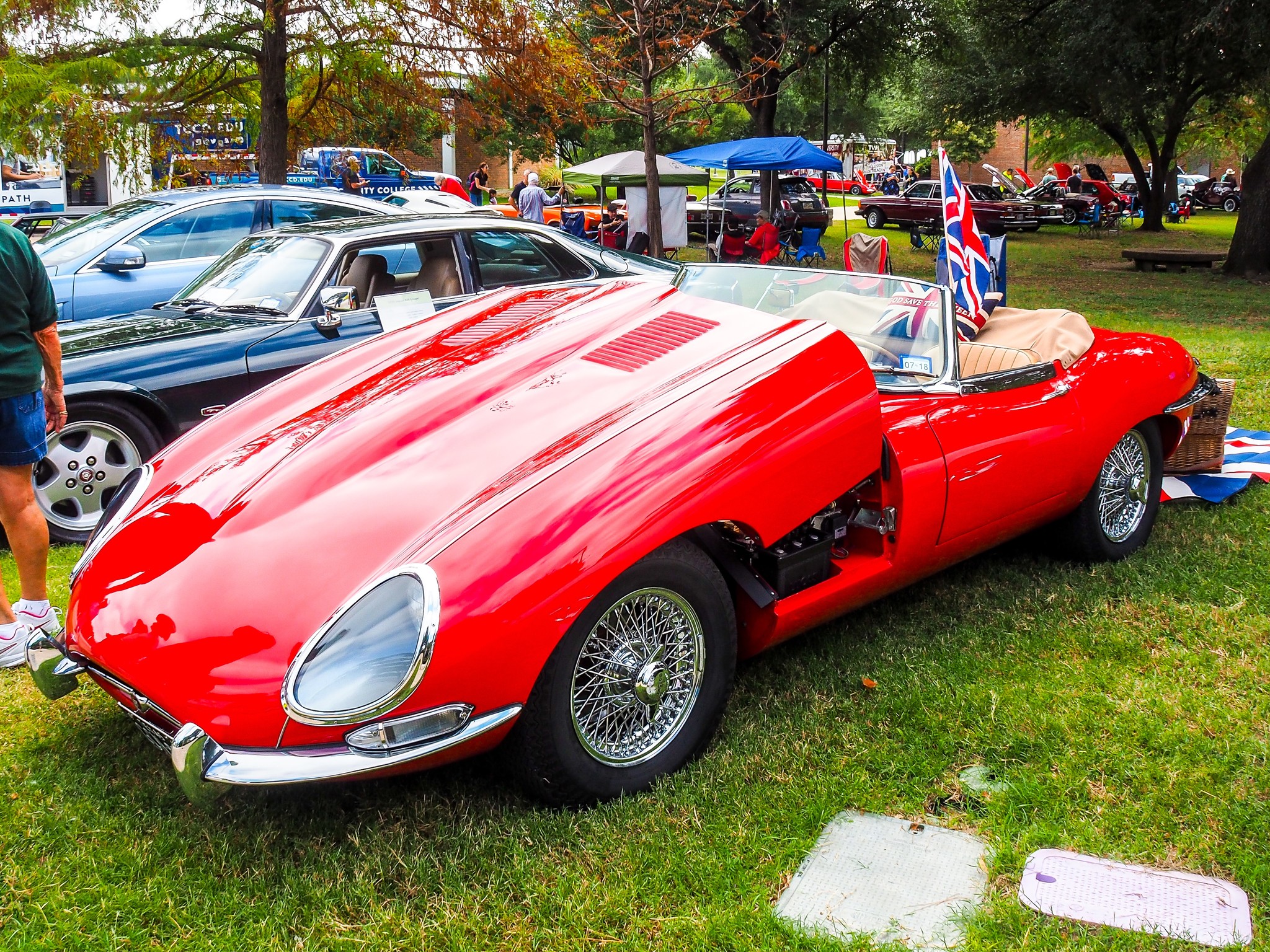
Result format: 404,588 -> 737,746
0,116 -> 152,222
198,147 -> 464,215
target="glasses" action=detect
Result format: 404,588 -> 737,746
890,169 -> 895,171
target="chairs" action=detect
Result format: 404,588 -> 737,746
913,342 -> 1036,385
562,211 -> 678,260
340,254 -> 395,303
843,233 -> 893,276
1076,196 -> 1140,239
1164,378 -> 1236,473
912,214 -> 945,255
707,208 -> 827,270
406,257 -> 462,298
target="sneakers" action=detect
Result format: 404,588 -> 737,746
11,602 -> 64,635
0,625 -> 45,667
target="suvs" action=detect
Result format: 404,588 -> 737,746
699,174 -> 829,235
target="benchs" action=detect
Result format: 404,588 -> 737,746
479,263 -> 553,285
777,288 -> 1095,374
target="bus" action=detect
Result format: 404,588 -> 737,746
785,134 -> 904,196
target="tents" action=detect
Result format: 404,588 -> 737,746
560,150 -> 709,262
666,137 -> 847,263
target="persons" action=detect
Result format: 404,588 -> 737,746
0,148 -> 43,190
489,189 -> 497,205
342,156 -> 368,196
708,210 -> 777,262
470,162 -> 491,206
434,175 -> 471,203
1066,165 -> 1082,194
0,223 -> 68,670
598,204 -> 625,233
1042,167 -> 1057,186
508,169 -> 532,218
883,165 -> 918,195
1221,168 -> 1237,188
993,168 -> 1013,192
518,172 -> 565,224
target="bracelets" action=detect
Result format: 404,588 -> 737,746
610,223 -> 613,227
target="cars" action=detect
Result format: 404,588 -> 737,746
853,162 -> 1241,238
31,209 -> 731,547
29,184 -> 519,325
381,190 -> 517,251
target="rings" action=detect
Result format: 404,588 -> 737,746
59,411 -> 68,415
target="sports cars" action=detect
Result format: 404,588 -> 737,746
480,185 -> 629,240
25,263 -> 1222,813
685,185 -> 732,238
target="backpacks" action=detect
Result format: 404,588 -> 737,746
466,171 -> 477,190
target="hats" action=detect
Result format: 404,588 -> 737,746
527,172 -> 539,182
347,156 -> 361,163
753,210 -> 769,219
1226,168 -> 1236,174
1045,167 -> 1054,176
607,204 -> 618,211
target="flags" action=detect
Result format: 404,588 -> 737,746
942,148 -> 990,314
